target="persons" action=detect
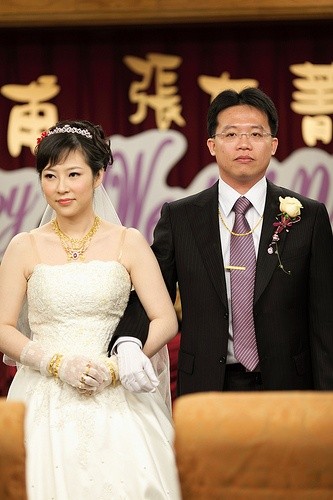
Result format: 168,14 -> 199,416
0,120 -> 179,500
109,87 -> 333,394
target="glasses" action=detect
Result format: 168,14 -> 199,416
212,130 -> 274,143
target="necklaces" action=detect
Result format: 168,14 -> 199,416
51,217 -> 101,261
218,209 -> 263,236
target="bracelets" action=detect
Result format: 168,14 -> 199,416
49,353 -> 63,377
106,361 -> 117,385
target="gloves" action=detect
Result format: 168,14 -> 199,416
118,342 -> 159,394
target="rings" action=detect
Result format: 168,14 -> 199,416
86,368 -> 90,376
81,375 -> 87,382
87,360 -> 92,367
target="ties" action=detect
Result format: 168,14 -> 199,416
230,197 -> 259,372
